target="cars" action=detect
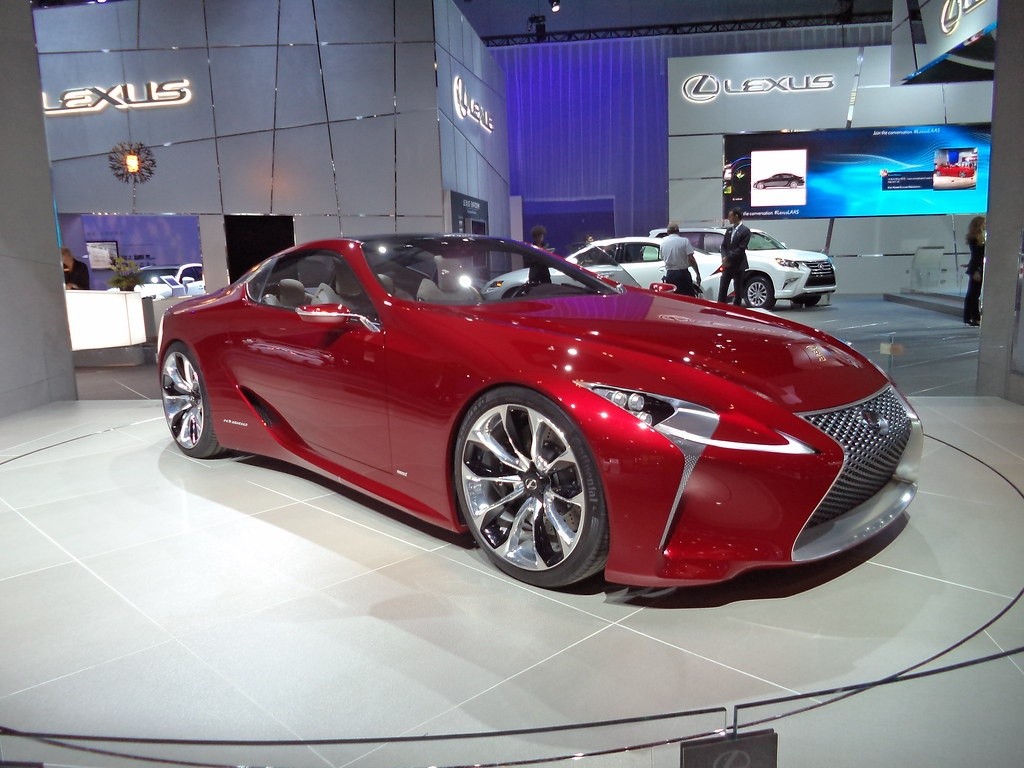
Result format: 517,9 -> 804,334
752,173 -> 804,190
479,235 -> 736,306
105,262 -> 206,299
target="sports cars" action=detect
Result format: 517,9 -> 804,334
154,230 -> 927,589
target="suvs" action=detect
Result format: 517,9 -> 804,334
642,225 -> 837,310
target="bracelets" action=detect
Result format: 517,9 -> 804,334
696,272 -> 700,276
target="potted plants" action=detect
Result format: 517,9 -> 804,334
106,253 -> 146,291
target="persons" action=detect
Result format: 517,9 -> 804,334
964,217 -> 986,326
659,223 -> 701,299
528,225 -> 552,284
61,247 -> 90,290
584,234 -> 594,248
717,207 -> 751,306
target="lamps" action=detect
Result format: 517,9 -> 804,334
527,1 -> 546,41
549,0 -> 560,12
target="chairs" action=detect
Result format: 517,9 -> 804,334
415,253 -> 484,304
309,259 -> 414,317
260,279 -> 314,310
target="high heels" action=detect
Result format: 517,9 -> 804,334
963,319 -> 980,326
